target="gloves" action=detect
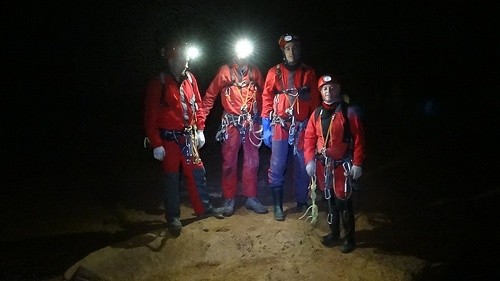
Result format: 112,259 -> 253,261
261,117 -> 272,149
305,161 -> 316,176
350,165 -> 362,179
197,129 -> 206,149
152,145 -> 166,162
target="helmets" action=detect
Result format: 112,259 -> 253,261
278,32 -> 305,48
317,73 -> 341,88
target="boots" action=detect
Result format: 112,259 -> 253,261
340,197 -> 355,254
318,190 -> 341,246
163,171 -> 183,231
187,167 -> 223,217
271,185 -> 286,221
295,203 -> 314,214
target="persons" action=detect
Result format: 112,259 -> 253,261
260,31 -> 322,219
197,37 -> 271,216
304,74 -> 367,252
143,38 -> 224,231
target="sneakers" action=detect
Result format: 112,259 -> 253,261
246,198 -> 266,214
221,197 -> 235,215
